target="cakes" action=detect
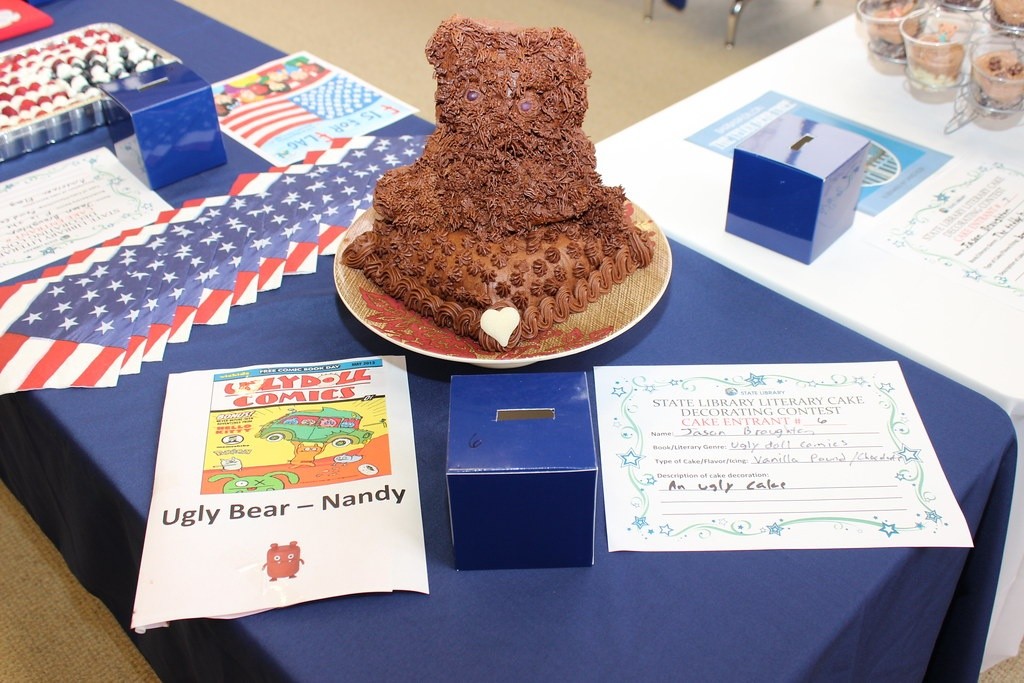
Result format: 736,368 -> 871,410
340,13 -> 654,351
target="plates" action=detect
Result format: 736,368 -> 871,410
334,201 -> 673,368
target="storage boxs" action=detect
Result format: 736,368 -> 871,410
98,61 -> 227,190
724,114 -> 871,265
444,374 -> 596,568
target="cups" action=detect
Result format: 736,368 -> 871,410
899,7 -> 977,104
856,0 -> 932,73
967,28 -> 1024,129
937,0 -> 983,20
990,0 -> 1024,40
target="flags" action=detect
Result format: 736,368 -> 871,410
0,131 -> 435,398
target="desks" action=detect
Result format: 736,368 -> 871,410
0,0 -> 1024,683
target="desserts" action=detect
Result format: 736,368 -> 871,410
858,0 -> 1024,130
0,28 -> 171,133
